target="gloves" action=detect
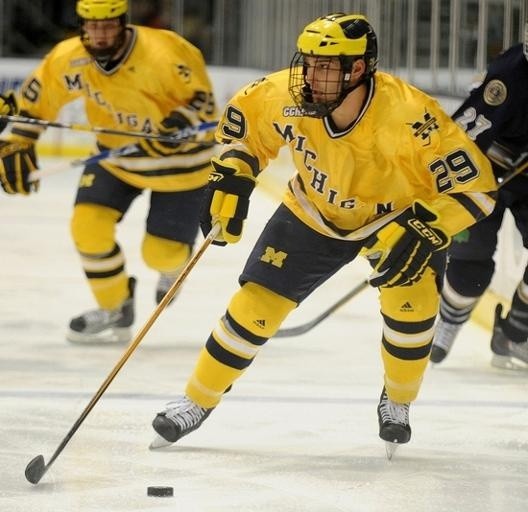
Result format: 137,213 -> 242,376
200,157 -> 258,246
360,199 -> 451,288
138,111 -> 195,158
0,126 -> 40,195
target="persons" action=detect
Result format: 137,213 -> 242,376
427,21 -> 527,366
1,1 -> 213,63
151,14 -> 503,445
0,1 -> 228,341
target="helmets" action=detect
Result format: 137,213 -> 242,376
76,0 -> 129,55
289,12 -> 378,118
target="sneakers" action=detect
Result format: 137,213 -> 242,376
156,272 -> 179,304
70,301 -> 135,334
378,385 -> 410,443
152,395 -> 214,442
430,318 -> 462,363
491,303 -> 528,363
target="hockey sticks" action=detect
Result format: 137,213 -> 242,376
24,221 -> 222,484
272,281 -> 371,336
